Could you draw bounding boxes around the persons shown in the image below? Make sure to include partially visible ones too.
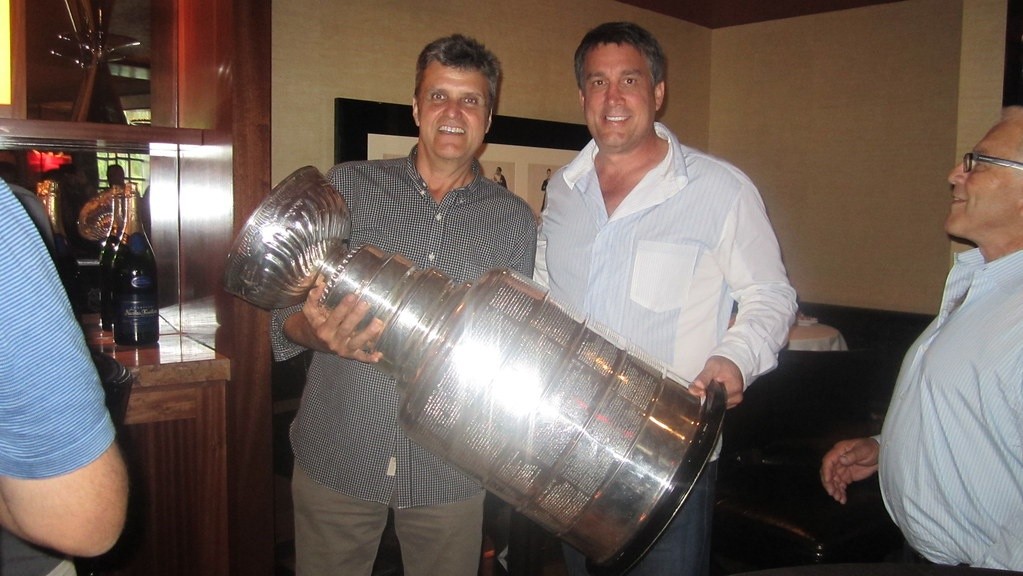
[106,163,127,189]
[822,104,1023,576]
[270,33,538,576]
[531,20,798,576]
[0,174,129,557]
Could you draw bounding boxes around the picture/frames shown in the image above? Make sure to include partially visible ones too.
[333,97,593,221]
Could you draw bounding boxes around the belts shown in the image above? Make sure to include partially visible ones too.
[903,542,932,564]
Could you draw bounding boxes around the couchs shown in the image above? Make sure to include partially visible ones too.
[710,301,953,573]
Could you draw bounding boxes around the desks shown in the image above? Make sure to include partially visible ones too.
[727,313,848,353]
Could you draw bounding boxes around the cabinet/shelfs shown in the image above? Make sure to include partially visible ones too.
[0,0,272,576]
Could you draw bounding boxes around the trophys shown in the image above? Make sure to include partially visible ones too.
[223,164,727,576]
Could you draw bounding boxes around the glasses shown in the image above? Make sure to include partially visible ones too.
[963,153,1023,174]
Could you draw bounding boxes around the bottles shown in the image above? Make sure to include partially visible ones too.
[44,181,80,323]
[113,182,160,346]
[101,185,124,332]
[37,183,45,208]
